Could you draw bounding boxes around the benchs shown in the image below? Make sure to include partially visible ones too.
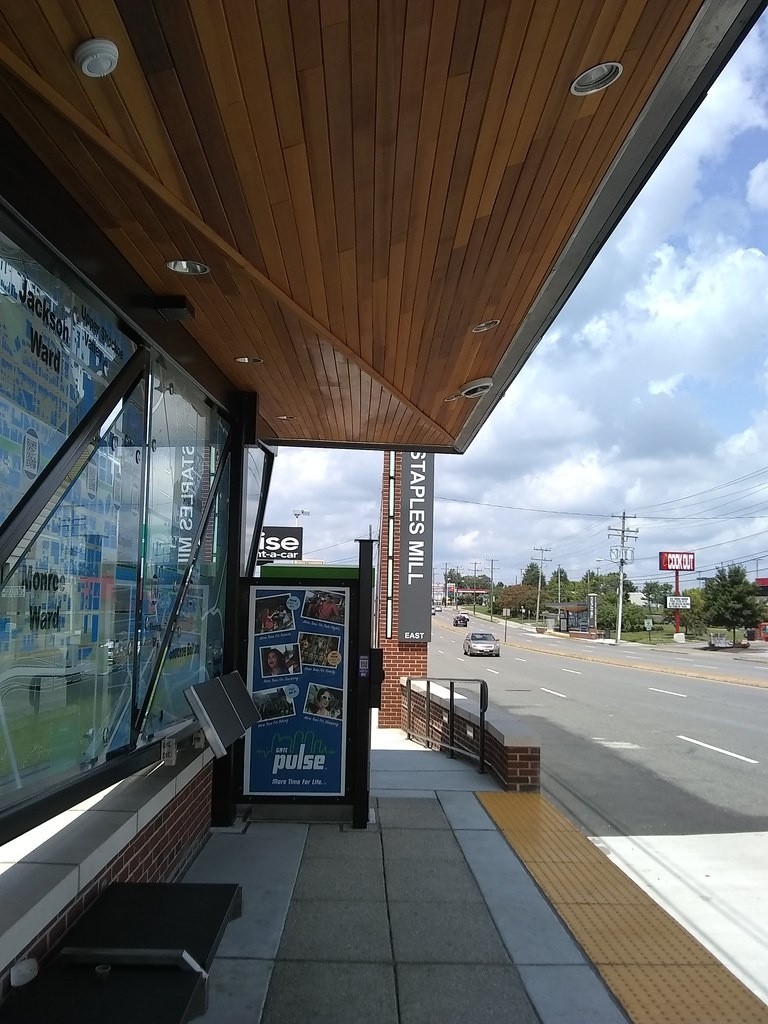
[0,881,244,1024]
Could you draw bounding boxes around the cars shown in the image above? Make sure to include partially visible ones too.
[453,611,469,626]
[463,633,501,657]
[431,605,442,615]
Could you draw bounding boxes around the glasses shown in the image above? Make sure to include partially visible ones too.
[320,695,332,701]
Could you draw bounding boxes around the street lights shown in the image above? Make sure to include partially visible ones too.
[596,559,624,643]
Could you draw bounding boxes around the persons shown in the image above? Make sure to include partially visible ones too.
[308,689,341,718]
[316,595,340,619]
[267,649,290,676]
[278,605,288,624]
[306,597,320,617]
[263,608,278,631]
